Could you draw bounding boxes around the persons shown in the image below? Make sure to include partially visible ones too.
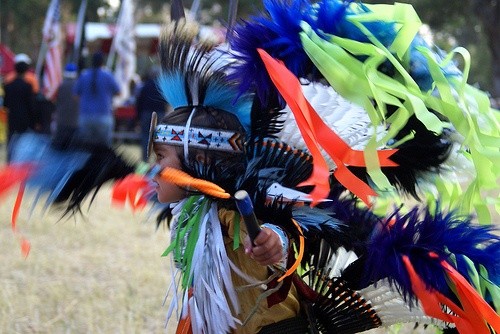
[52,63,82,148]
[75,52,120,146]
[3,53,44,165]
[148,104,315,333]
